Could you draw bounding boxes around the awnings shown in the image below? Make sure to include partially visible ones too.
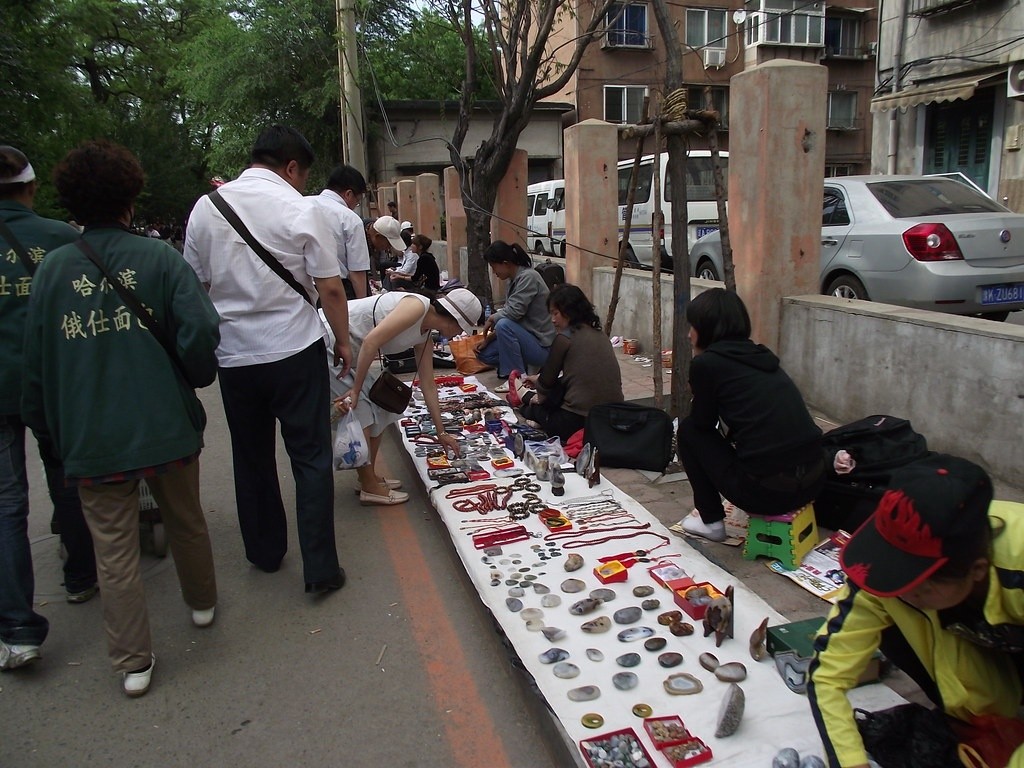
[869,69,1008,113]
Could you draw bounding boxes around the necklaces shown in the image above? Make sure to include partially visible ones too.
[544,487,672,549]
[501,490,512,510]
[493,493,500,510]
[445,484,497,514]
[460,516,519,533]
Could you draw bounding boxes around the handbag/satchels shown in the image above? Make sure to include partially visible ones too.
[448,333,495,376]
[369,371,412,414]
[333,407,371,471]
[582,401,673,473]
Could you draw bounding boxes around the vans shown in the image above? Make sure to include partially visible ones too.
[546,150,731,268]
[526,179,565,257]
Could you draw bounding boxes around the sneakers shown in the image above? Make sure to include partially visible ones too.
[0,639,41,669]
[66,582,98,603]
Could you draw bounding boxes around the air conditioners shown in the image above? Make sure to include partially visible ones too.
[704,49,726,69]
[868,42,877,56]
[1007,64,1024,97]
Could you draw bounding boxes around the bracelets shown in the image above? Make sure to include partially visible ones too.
[529,504,548,513]
[507,477,544,512]
[509,510,530,520]
[493,486,508,494]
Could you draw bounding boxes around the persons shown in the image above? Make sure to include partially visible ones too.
[474,241,557,391]
[681,287,824,539]
[507,282,624,441]
[0,146,98,666]
[137,216,188,254]
[319,287,483,505]
[304,165,370,301]
[812,452,1024,768]
[184,124,350,591]
[29,143,220,697]
[363,202,440,303]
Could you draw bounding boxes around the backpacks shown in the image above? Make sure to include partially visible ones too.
[817,414,941,499]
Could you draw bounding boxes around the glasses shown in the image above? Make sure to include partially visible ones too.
[347,188,361,207]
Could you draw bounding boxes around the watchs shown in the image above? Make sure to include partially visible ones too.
[437,431,448,438]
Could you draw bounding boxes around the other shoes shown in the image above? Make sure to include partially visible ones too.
[494,384,510,393]
[192,606,215,627]
[123,652,156,695]
[359,489,409,505]
[506,369,521,407]
[355,476,401,495]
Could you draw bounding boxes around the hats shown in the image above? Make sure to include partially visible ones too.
[400,221,413,231]
[436,288,482,337]
[373,216,407,251]
[839,456,994,598]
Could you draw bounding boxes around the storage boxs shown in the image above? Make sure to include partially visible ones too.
[673,581,725,620]
[491,456,514,470]
[644,715,692,751]
[593,560,628,585]
[662,736,713,768]
[427,457,450,469]
[462,384,479,392]
[539,512,572,533]
[580,727,657,768]
[766,617,886,689]
[401,420,422,438]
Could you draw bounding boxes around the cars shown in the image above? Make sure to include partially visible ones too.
[690,172,1024,324]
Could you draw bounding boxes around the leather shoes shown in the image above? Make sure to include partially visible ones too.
[306,567,345,593]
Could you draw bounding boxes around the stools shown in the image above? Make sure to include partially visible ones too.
[742,502,819,571]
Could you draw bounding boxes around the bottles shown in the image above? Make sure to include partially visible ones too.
[330,397,351,424]
[441,334,448,345]
[485,305,491,321]
